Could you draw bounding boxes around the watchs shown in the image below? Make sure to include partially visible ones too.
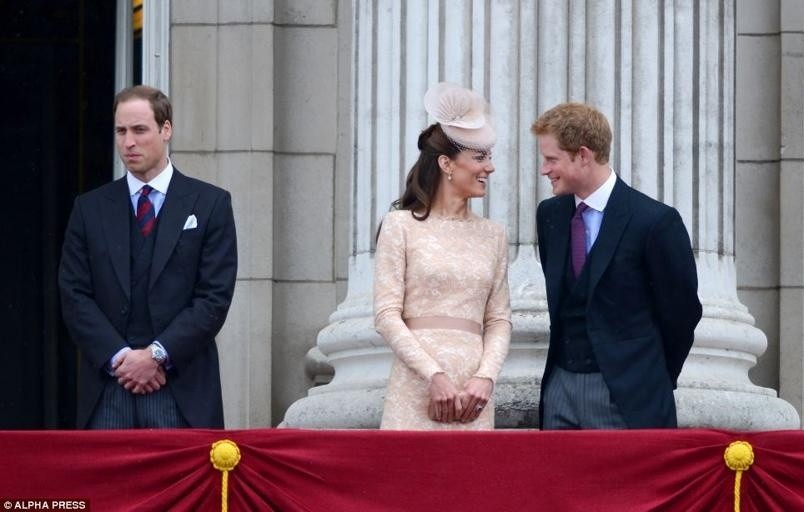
[148,342,165,365]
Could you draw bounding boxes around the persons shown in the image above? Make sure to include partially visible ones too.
[529,101,702,428]
[56,87,238,430]
[371,82,513,430]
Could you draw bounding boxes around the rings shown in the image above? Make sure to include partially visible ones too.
[475,403,482,413]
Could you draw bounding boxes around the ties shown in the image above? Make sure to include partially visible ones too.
[136,185,157,239]
[569,201,588,281]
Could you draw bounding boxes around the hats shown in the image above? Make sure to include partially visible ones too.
[440,115,498,153]
